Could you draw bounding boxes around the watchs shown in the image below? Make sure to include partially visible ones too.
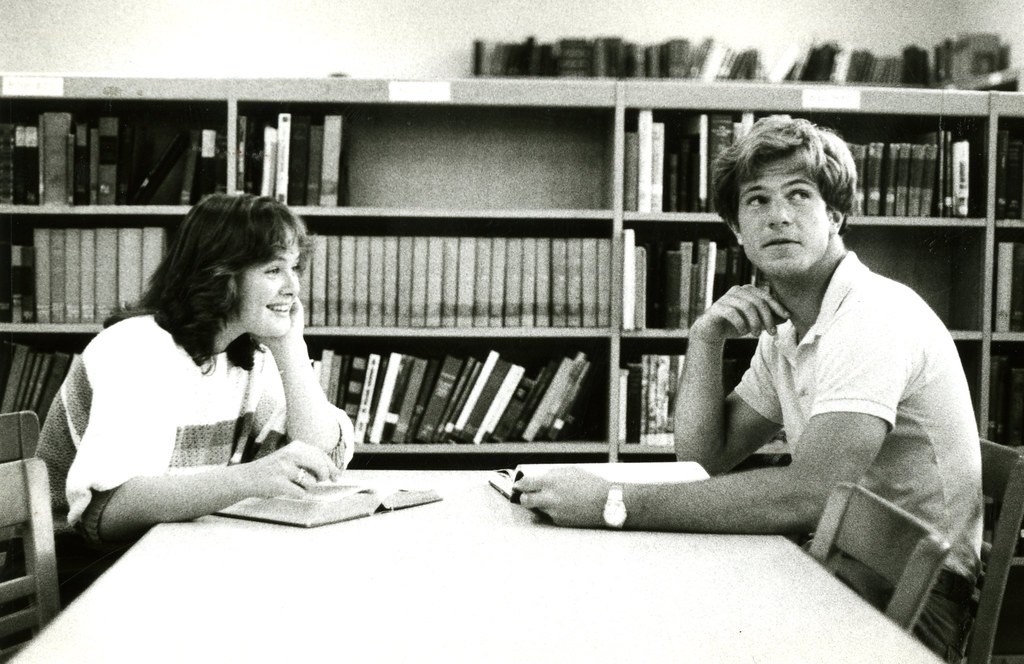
[603,485,628,528]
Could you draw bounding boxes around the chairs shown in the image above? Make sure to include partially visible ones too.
[0,410,64,662]
[806,435,1024,660]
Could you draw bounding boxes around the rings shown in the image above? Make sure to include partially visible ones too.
[295,469,309,484]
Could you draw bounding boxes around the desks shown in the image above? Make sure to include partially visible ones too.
[4,460,943,663]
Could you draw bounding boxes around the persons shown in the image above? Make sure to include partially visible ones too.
[0,192,357,646]
[513,111,985,664]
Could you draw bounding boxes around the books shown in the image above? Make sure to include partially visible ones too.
[0,33,1024,441]
[212,482,441,529]
[486,461,711,500]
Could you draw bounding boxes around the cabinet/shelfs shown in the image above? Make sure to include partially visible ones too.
[0,72,1024,647]
